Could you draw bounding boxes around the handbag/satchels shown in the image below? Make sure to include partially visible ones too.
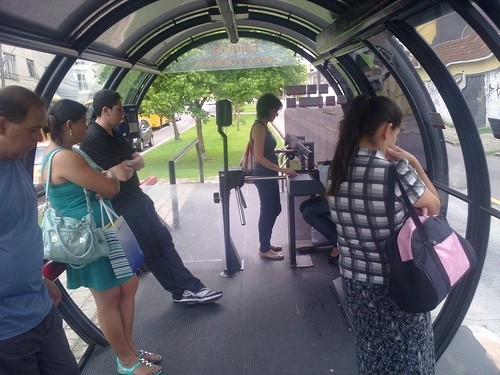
[385,161,479,313]
[239,125,257,184]
[40,151,109,264]
[98,197,146,280]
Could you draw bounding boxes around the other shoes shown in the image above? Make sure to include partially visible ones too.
[259,250,284,260]
[328,251,339,264]
[271,246,282,251]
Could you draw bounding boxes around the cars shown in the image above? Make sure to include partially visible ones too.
[131,119,154,152]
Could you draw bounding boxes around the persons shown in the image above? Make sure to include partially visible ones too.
[250,93,297,260]
[325,94,441,375]
[42,99,163,375]
[299,161,339,261]
[0,85,81,375]
[78,89,223,304]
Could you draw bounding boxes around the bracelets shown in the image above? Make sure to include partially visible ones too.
[41,274,46,279]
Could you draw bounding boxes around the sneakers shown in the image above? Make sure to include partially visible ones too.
[173,286,223,304]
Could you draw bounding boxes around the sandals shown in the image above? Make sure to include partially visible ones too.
[116,348,163,375]
